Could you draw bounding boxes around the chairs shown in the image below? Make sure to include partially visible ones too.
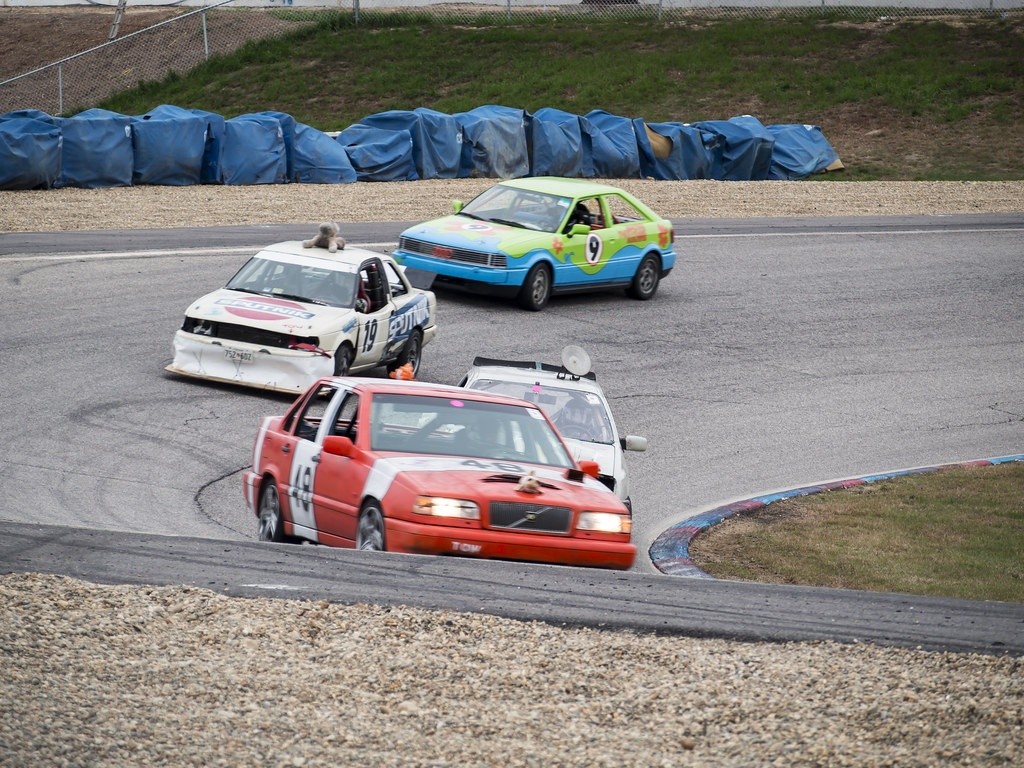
[556,398,597,439]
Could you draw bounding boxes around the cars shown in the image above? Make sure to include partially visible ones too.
[242,376,637,571]
[418,356,648,520]
[164,241,438,400]
[390,176,677,310]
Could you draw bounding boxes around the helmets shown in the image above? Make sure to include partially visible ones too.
[565,204,590,230]
[466,419,500,445]
[335,270,362,289]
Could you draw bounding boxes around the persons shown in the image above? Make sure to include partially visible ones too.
[460,417,518,458]
[324,273,371,313]
[550,200,591,233]
[554,399,596,442]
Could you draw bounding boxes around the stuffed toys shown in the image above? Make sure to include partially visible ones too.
[303,221,345,253]
